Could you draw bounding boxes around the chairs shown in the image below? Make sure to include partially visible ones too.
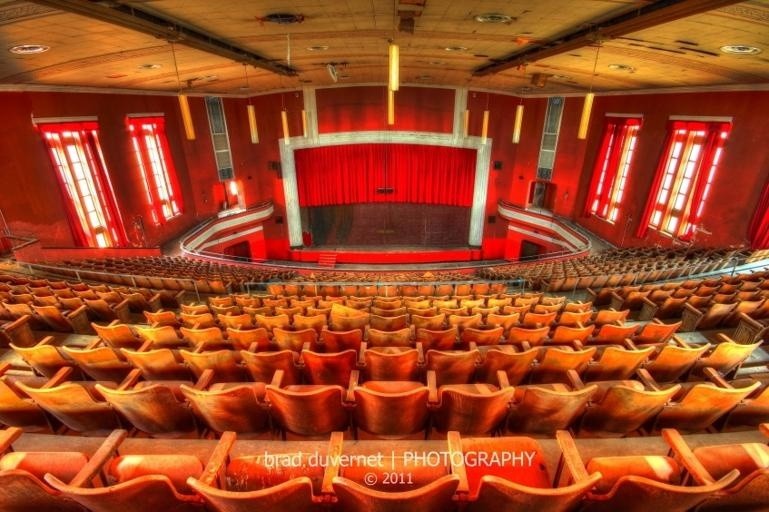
[693,423,769,512]
[1,426,128,512]
[44,430,238,512]
[331,431,469,511]
[460,429,602,511]
[583,428,741,512]
[186,430,344,511]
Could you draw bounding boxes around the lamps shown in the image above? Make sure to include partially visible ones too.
[280,78,290,145]
[387,91,395,125]
[577,46,602,139]
[511,66,527,144]
[243,64,260,145]
[463,110,470,137]
[169,42,197,140]
[480,73,492,144]
[389,44,400,91]
